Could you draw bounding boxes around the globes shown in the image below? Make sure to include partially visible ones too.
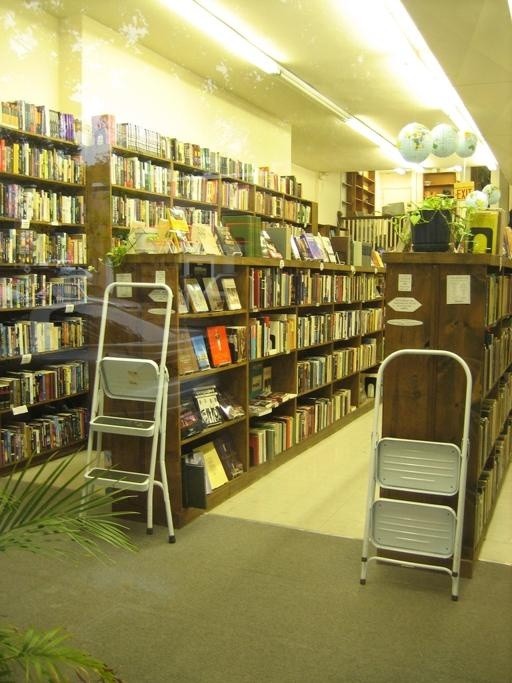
[397,122,477,163]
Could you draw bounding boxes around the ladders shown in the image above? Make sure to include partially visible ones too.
[76,282,176,543]
[361,348,472,601]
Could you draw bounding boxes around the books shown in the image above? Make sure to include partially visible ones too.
[1,100,89,462]
[474,271,512,556]
[177,215,386,509]
[462,206,507,258]
[112,123,312,249]
